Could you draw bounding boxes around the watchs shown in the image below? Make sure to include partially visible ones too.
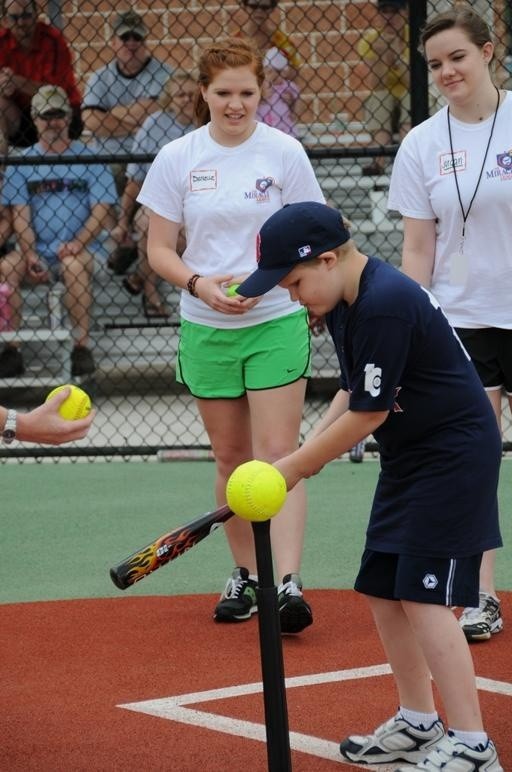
[2,409,17,439]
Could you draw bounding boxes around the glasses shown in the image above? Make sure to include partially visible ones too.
[119,32,142,41]
[40,111,65,120]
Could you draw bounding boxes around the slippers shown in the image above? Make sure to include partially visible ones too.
[109,246,168,317]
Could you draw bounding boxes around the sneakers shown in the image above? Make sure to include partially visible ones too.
[277,574,313,633]
[0,346,25,378]
[71,345,96,384]
[458,592,503,640]
[213,567,258,622]
[340,713,504,772]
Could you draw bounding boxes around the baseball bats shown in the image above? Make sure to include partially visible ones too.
[110,503,235,590]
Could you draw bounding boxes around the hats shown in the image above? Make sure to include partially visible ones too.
[112,11,147,38]
[31,85,73,116]
[235,201,350,298]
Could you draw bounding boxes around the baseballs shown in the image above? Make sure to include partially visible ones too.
[227,284,241,297]
[226,460,286,521]
[46,384,91,419]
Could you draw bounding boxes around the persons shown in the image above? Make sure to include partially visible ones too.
[0,385,97,445]
[236,202,504,772]
[356,2,412,176]
[387,6,511,643]
[0,0,307,384]
[136,39,328,632]
